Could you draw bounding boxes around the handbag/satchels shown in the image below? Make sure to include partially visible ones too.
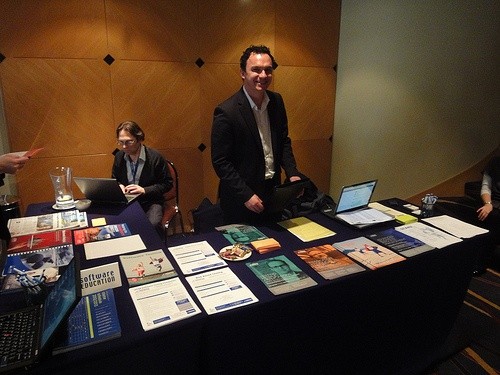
[192,197,226,233]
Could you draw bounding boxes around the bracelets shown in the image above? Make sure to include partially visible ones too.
[484,201,491,204]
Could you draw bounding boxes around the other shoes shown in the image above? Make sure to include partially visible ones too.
[473,270,478,277]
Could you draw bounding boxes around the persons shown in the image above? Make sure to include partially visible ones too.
[111,120,174,242]
[464,155,500,277]
[266,259,309,288]
[354,247,368,256]
[210,45,305,227]
[361,243,391,257]
[131,261,145,278]
[0,151,31,188]
[219,225,250,242]
[150,257,164,272]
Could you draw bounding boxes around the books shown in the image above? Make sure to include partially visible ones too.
[0,202,489,356]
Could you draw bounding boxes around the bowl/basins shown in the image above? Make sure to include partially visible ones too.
[73,200,91,210]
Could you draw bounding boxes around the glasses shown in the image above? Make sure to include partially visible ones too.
[117,139,137,145]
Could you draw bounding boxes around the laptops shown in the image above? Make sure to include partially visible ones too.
[73,177,141,207]
[334,179,395,230]
[0,253,82,374]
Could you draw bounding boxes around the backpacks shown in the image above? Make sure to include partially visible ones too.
[281,172,335,222]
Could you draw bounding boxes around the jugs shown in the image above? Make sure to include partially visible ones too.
[49,167,74,207]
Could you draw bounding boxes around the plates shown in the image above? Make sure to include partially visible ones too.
[52,200,79,210]
[219,246,252,261]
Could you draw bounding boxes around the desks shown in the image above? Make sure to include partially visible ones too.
[22,195,479,375]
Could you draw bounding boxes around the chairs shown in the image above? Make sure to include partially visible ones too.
[154,159,184,250]
